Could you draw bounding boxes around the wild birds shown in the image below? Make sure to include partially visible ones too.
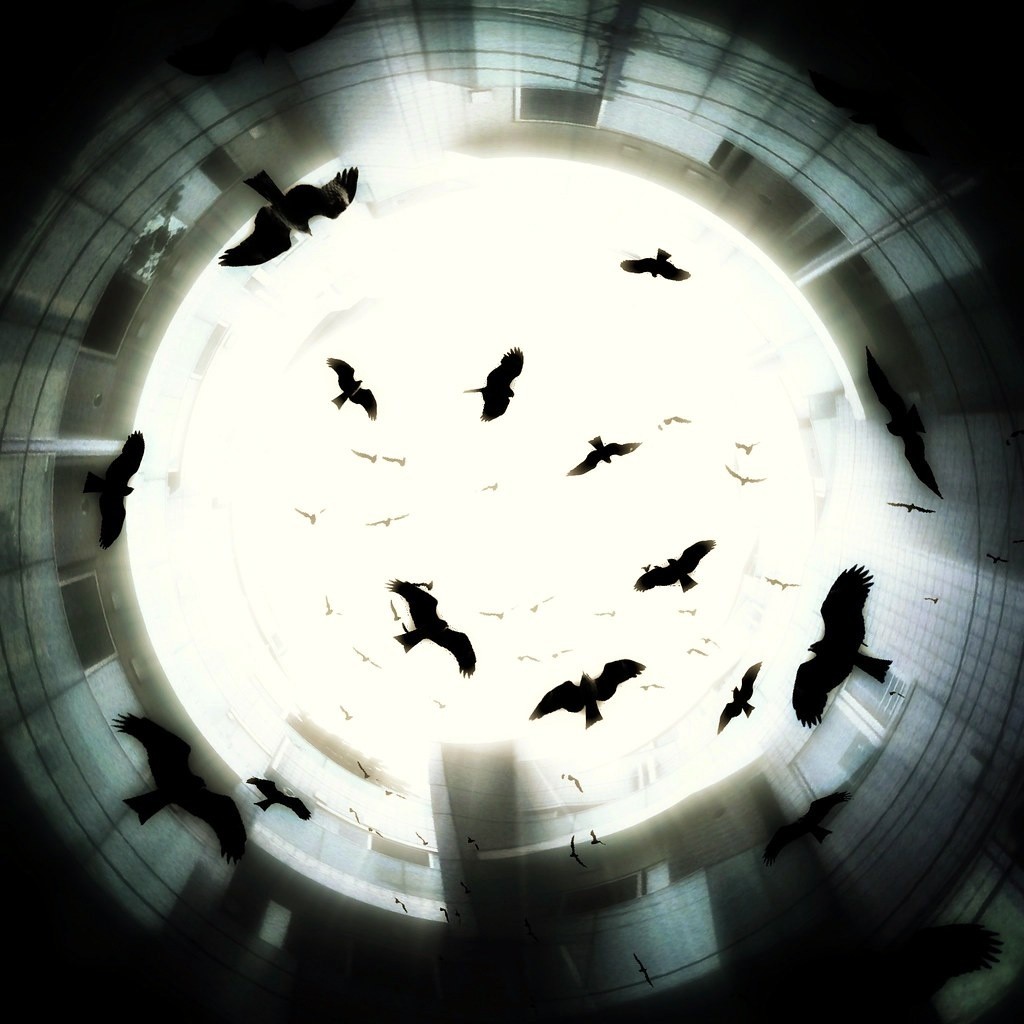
[81,167,1019,942]
